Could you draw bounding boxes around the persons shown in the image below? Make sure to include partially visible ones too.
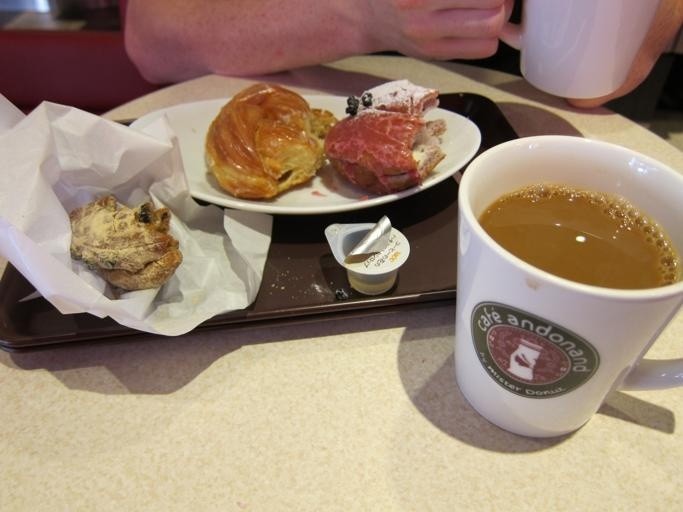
[119,0,683,108]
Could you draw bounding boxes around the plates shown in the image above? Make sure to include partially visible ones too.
[122,92,484,219]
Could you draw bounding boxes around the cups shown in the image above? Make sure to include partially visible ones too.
[452,135,683,444]
[496,1,665,104]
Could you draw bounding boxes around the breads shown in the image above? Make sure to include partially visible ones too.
[204,83,337,200]
[71,196,181,291]
[324,76,446,194]
